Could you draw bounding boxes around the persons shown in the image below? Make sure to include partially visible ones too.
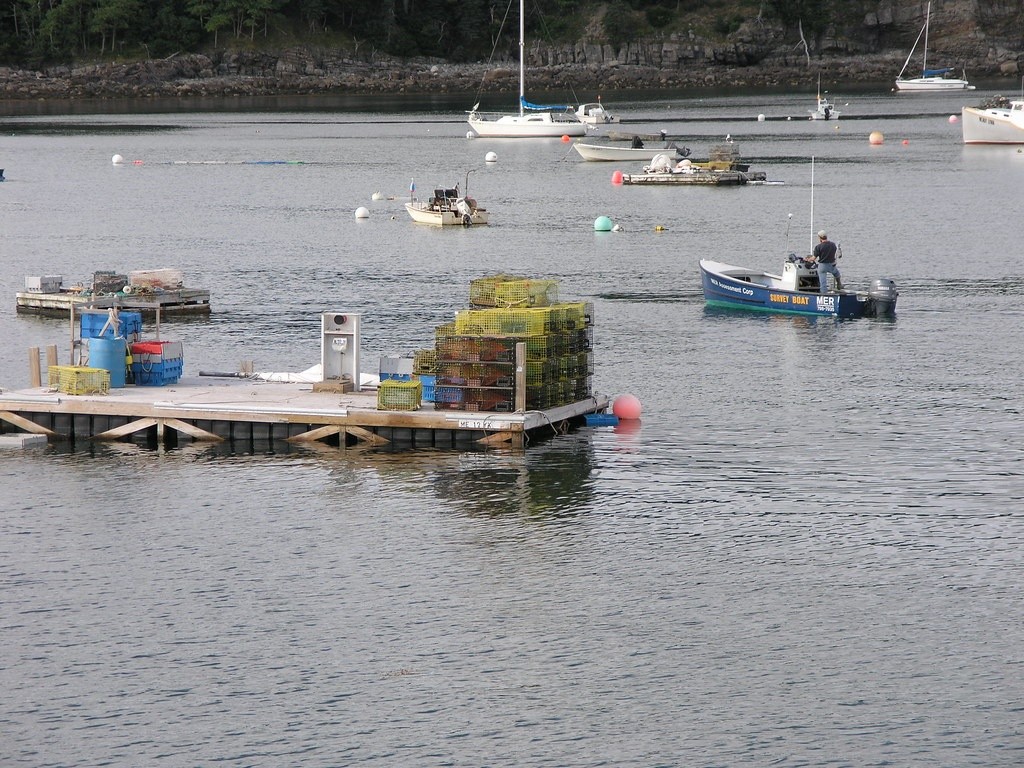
[803,230,844,293]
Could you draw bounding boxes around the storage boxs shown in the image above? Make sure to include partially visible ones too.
[375,273,594,412]
[47,364,112,395]
[130,341,183,387]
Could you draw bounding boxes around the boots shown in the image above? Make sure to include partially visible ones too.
[836,277,844,290]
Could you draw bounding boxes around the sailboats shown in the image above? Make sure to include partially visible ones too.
[463,0,590,138]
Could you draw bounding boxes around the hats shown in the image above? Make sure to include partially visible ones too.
[818,230,827,238]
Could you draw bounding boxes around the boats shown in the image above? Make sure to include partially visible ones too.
[697,155,898,321]
[961,98,1024,144]
[573,138,693,161]
[690,157,751,172]
[894,1,977,90]
[574,100,620,124]
[812,97,841,121]
[605,128,667,140]
[403,178,490,227]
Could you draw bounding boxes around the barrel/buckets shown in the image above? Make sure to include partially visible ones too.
[89,335,126,388]
